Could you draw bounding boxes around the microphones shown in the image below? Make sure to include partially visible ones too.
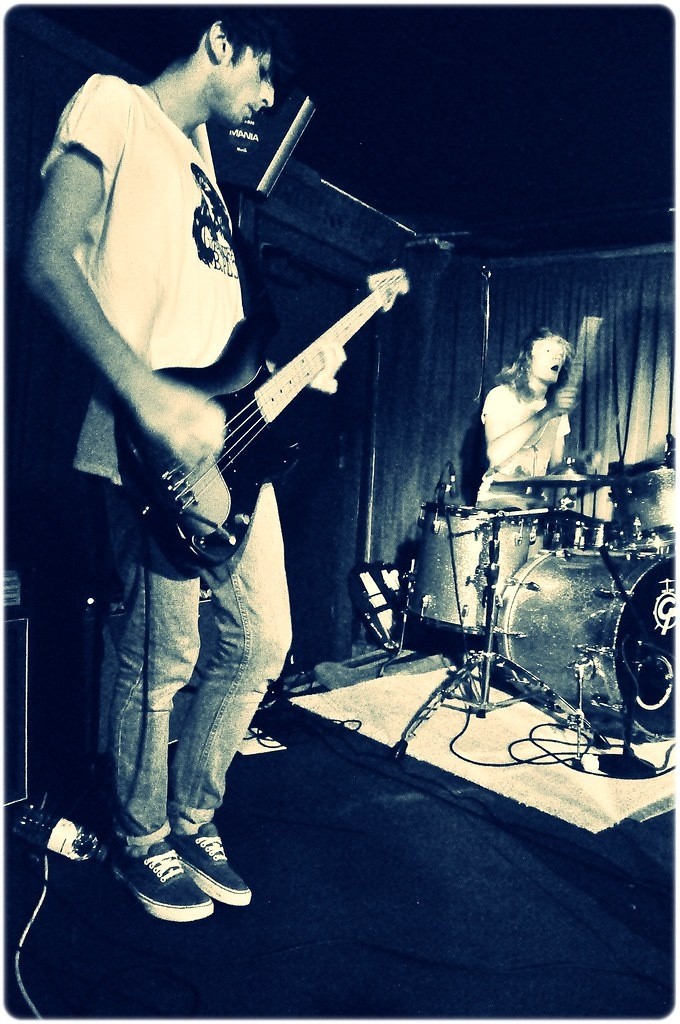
[448,463,458,499]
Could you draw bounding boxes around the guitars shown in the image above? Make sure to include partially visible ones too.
[121,267,410,571]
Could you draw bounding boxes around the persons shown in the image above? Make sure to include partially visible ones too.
[479,327,603,511]
[24,4,345,923]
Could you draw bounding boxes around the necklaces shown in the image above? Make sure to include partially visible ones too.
[145,82,164,112]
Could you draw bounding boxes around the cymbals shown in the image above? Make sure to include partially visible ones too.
[489,468,619,490]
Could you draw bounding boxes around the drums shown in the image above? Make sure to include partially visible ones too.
[496,545,675,743]
[610,467,675,553]
[408,500,533,642]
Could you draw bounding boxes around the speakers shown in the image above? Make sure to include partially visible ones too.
[4,604,38,808]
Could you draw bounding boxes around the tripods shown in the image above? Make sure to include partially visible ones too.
[388,482,610,767]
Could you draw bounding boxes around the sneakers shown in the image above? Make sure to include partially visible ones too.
[169,822,254,907]
[112,842,215,924]
